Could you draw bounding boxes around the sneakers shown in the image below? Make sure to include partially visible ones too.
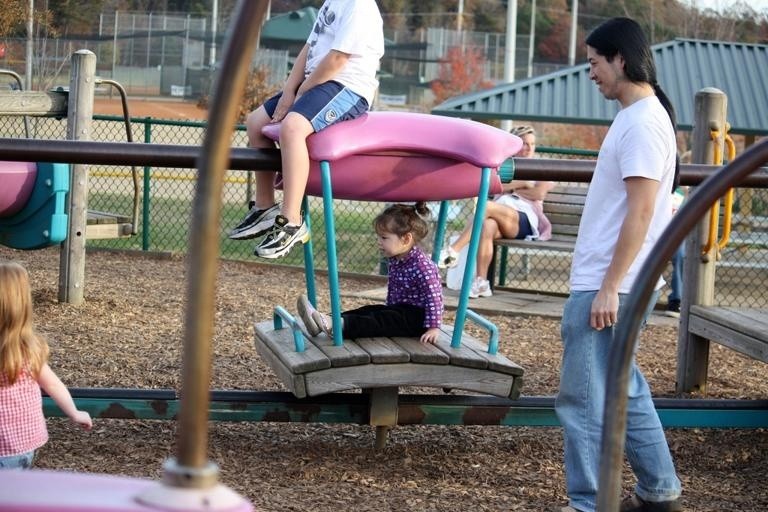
[427,244,462,268]
[618,494,687,512]
[227,202,281,242]
[295,292,342,340]
[254,211,313,261]
[466,275,492,298]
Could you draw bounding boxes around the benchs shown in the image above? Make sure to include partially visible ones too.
[486,181,588,293]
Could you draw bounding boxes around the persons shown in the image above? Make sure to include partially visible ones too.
[438,126,552,299]
[1,260,93,469]
[552,16,683,512]
[668,189,685,311]
[226,0,386,261]
[297,202,445,344]
